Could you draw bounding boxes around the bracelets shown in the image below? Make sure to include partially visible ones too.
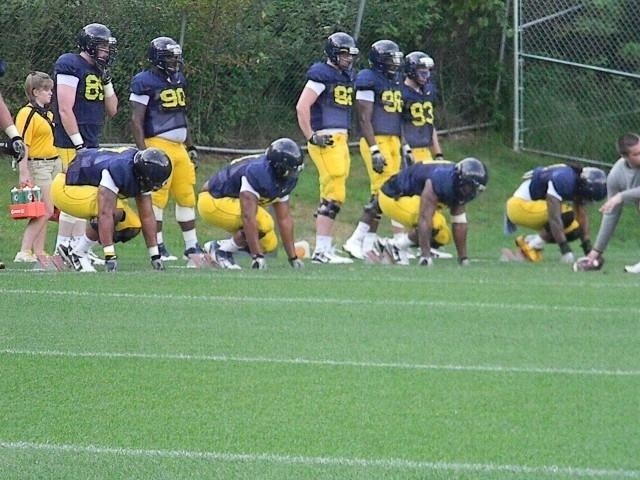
[558,243,571,253]
[582,240,592,254]
[592,248,603,253]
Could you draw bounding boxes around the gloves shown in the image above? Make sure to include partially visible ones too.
[0,138,15,158]
[401,150,416,169]
[185,145,202,170]
[4,135,29,164]
[248,253,270,272]
[95,56,113,86]
[457,256,473,267]
[578,239,605,271]
[418,256,436,268]
[434,153,447,162]
[371,149,388,174]
[559,241,576,266]
[287,255,307,271]
[148,254,166,271]
[308,131,337,149]
[72,143,88,153]
[102,252,120,274]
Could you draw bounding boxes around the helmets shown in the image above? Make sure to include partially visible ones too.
[454,156,488,192]
[323,30,361,60]
[146,34,185,66]
[76,22,119,52]
[134,147,173,193]
[402,50,436,74]
[580,164,608,202]
[367,37,405,69]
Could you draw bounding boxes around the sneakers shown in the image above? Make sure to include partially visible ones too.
[65,249,99,275]
[623,259,640,276]
[310,247,358,267]
[11,248,40,264]
[0,259,6,270]
[52,249,67,265]
[416,245,455,260]
[33,251,53,267]
[342,237,417,268]
[157,241,179,265]
[86,247,107,268]
[56,240,73,260]
[183,242,214,270]
[211,247,246,273]
[203,239,222,257]
[514,234,544,263]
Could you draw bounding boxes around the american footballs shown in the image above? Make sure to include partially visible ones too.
[573,256,604,272]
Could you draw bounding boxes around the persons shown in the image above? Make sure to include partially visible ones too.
[575,132,640,273]
[506,162,606,270]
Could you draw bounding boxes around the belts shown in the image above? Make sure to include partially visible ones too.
[26,154,63,162]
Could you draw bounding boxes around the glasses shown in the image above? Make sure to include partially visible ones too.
[264,136,305,173]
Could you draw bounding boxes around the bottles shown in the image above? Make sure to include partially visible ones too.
[11,185,41,204]
